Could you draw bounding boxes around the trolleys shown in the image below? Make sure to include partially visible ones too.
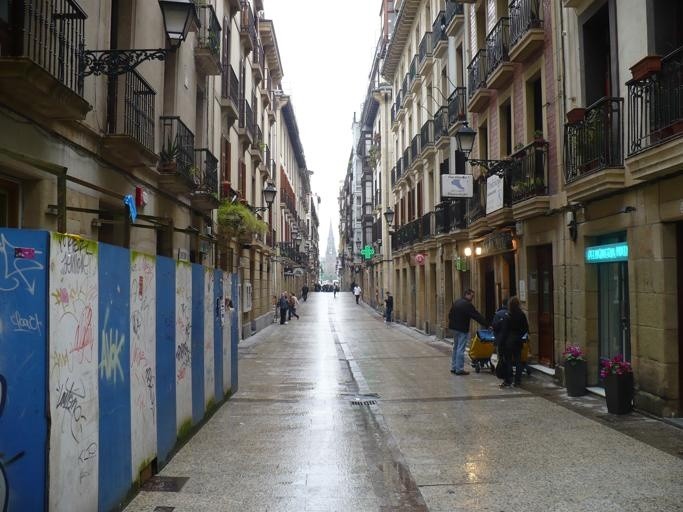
[468,327,495,373]
[512,330,532,376]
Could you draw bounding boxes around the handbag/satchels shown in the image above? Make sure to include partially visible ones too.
[520,336,529,362]
[495,352,513,379]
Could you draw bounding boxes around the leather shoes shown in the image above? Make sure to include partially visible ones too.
[456,370,469,375]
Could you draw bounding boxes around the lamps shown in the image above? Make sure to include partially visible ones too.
[383,207,415,230]
[274,226,302,250]
[246,181,277,214]
[82,0,197,88]
[455,121,522,178]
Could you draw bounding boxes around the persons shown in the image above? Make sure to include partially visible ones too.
[382,291,393,324]
[447,289,487,376]
[491,297,530,377]
[301,280,362,305]
[489,296,529,388]
[276,290,300,325]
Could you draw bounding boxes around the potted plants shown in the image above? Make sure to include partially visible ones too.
[218,203,270,247]
[160,134,181,174]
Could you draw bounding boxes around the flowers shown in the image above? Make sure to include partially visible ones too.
[600,354,632,377]
[561,344,587,362]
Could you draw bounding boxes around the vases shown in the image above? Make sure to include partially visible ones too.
[564,360,587,396]
[601,374,634,414]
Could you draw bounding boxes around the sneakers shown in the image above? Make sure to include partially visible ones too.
[499,382,510,389]
